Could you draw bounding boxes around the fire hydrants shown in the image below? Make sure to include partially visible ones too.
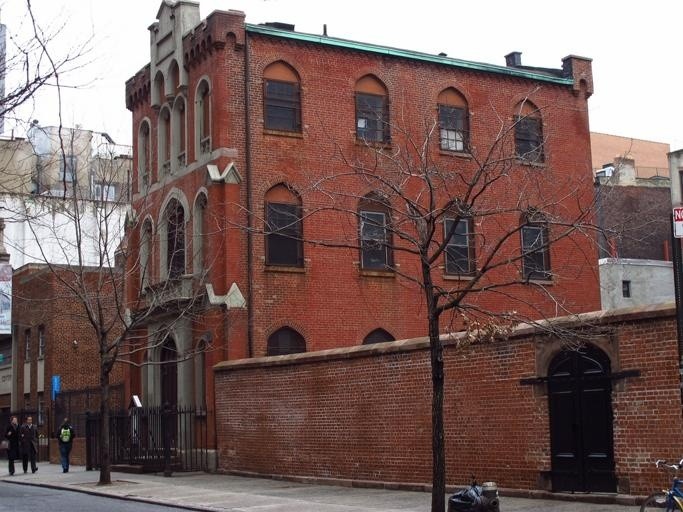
[473,482,500,512]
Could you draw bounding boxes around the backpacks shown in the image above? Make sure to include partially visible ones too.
[60,428,70,442]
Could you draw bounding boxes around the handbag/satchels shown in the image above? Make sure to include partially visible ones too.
[0,440,10,450]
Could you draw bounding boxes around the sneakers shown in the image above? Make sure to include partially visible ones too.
[32,467,38,473]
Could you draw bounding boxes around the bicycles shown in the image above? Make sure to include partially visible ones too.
[640,459,683,512]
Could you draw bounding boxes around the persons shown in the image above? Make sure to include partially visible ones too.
[5,416,24,475]
[20,416,39,473]
[56,417,75,473]
